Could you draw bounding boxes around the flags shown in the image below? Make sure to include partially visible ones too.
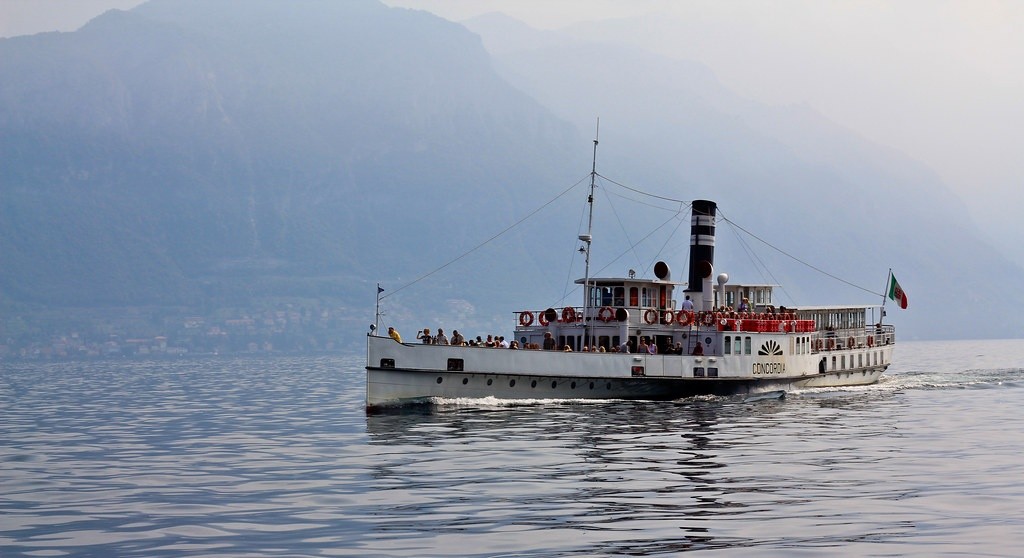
[888,272,908,310]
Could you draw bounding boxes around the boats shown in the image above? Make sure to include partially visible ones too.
[364,116,896,412]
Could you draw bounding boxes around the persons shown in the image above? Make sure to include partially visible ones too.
[714,298,797,320]
[388,327,402,343]
[584,338,704,356]
[682,295,694,311]
[418,328,571,351]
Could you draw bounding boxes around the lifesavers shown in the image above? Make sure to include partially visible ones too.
[723,312,730,318]
[792,313,798,320]
[599,306,614,322]
[811,340,814,349]
[775,313,790,320]
[734,312,740,319]
[715,312,723,325]
[663,311,674,324]
[849,337,855,347]
[520,311,534,326]
[816,339,823,352]
[741,312,758,319]
[562,307,575,323]
[677,310,690,326]
[702,311,715,326]
[644,309,658,325]
[759,312,774,320]
[868,336,873,347]
[828,339,834,350]
[539,312,549,326]
